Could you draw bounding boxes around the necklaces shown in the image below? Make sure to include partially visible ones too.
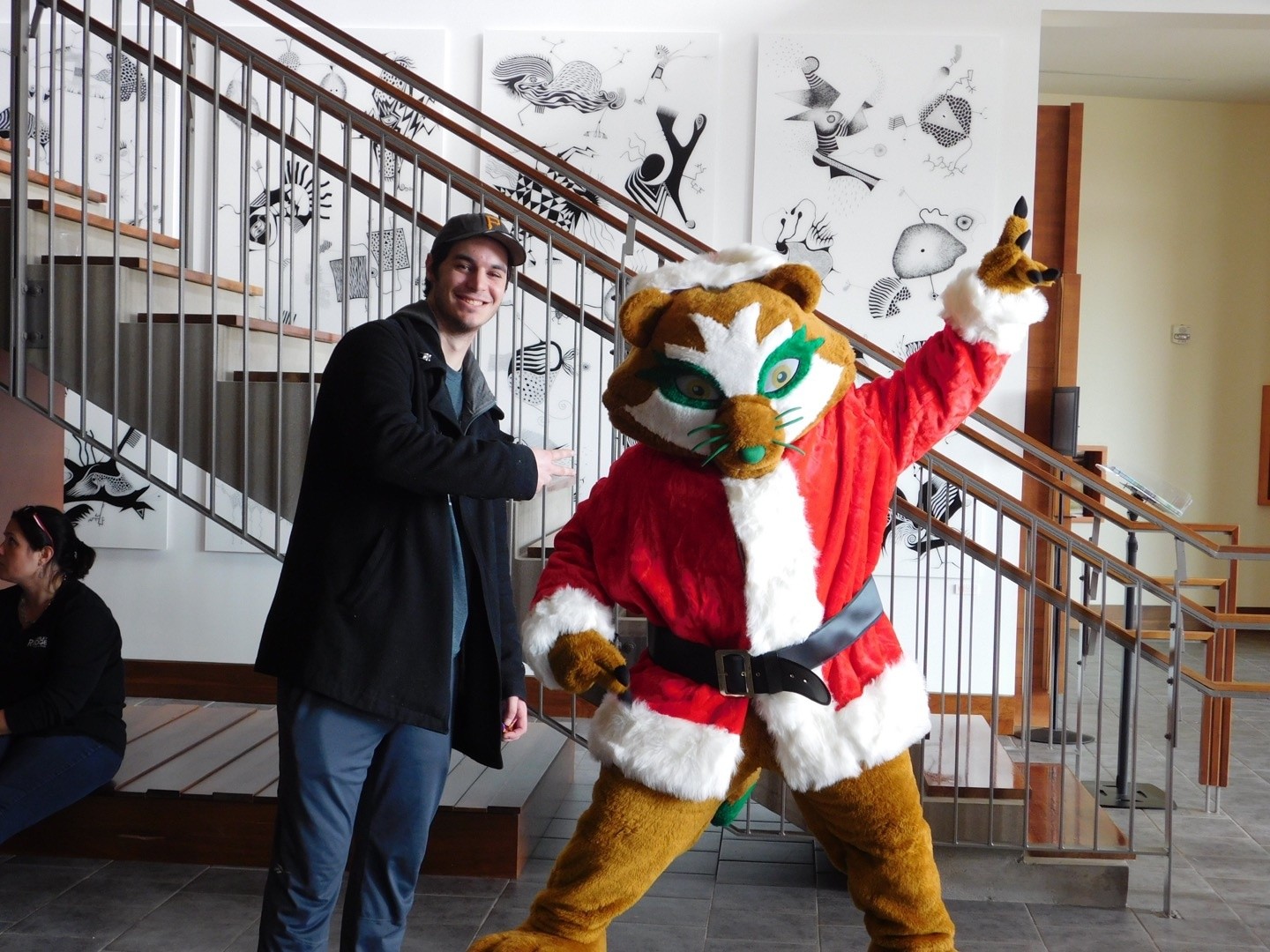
[18,573,67,626]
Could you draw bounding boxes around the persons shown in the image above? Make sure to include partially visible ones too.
[0,502,127,845]
[258,211,598,951]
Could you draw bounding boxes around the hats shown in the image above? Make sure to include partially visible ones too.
[432,212,527,266]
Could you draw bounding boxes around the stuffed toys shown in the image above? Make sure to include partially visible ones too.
[468,193,1062,951]
